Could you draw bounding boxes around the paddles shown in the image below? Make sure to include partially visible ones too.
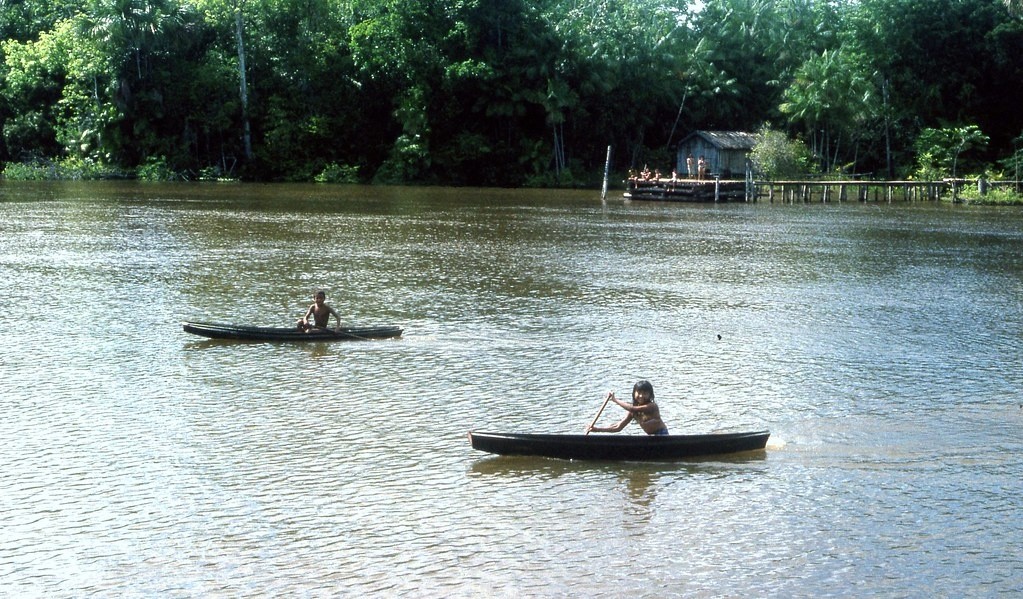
[584,392,614,436]
[300,324,371,341]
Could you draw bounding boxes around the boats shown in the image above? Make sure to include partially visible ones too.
[185,321,404,341]
[467,431,773,458]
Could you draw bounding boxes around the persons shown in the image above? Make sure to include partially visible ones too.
[687,154,697,181]
[698,155,706,181]
[666,168,681,192]
[628,163,662,189]
[587,380,668,436]
[296,291,342,335]
